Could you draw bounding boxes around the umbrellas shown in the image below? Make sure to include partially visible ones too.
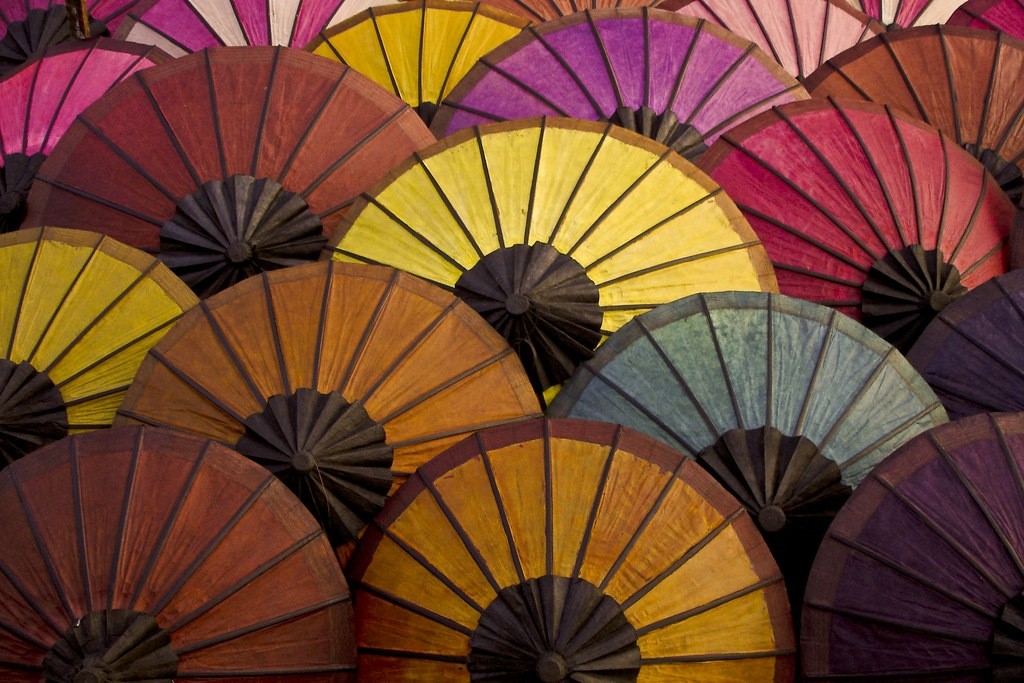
[0,1,1024,612]
[0,426,362,683]
[800,410,1024,683]
[343,416,797,683]
